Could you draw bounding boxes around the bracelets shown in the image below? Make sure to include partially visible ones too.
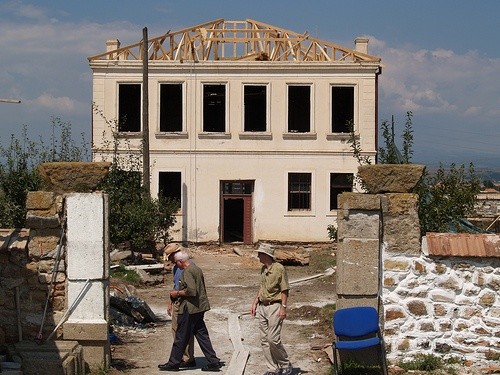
[177,291,179,295]
[283,305,286,307]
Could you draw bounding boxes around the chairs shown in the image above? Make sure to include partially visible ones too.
[333,307,388,375]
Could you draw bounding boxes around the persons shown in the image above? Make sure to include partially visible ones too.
[164,243,196,367]
[251,243,293,375]
[158,252,222,371]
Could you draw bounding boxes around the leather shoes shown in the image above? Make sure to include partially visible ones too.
[202,361,222,371]
[158,363,179,370]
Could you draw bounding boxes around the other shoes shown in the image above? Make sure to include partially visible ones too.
[179,359,196,368]
[278,362,293,375]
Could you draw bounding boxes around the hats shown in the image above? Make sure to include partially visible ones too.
[255,243,275,259]
[164,243,183,261]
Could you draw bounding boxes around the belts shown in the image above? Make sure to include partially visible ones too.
[259,300,282,304]
